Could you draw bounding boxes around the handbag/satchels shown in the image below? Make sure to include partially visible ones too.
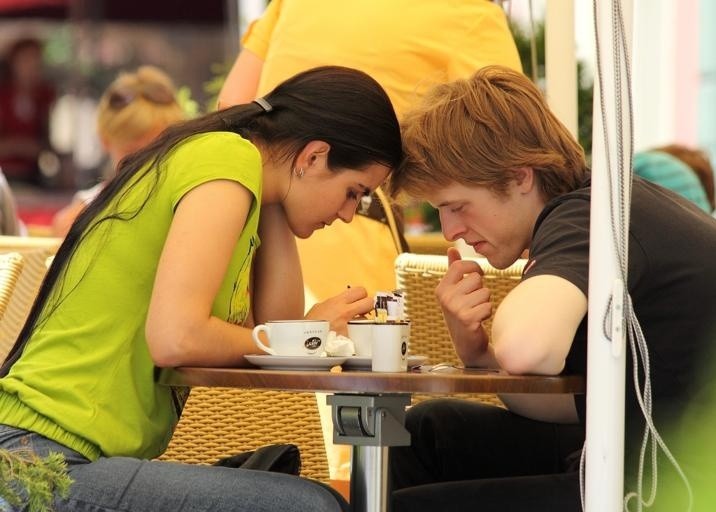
[213,444,300,475]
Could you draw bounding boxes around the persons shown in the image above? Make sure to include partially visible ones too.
[632,138,714,218]
[0,32,58,187]
[50,63,193,236]
[383,62,714,511]
[211,1,522,504]
[0,63,404,512]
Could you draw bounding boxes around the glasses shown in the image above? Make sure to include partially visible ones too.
[110,83,172,107]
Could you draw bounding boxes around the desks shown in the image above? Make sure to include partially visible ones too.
[156,367,586,512]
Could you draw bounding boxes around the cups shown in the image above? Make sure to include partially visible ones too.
[371,322,410,374]
[347,319,378,356]
[251,318,330,356]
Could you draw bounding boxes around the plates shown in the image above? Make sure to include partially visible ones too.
[341,355,428,371]
[243,353,349,371]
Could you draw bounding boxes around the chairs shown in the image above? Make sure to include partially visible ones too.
[0,235,330,487]
[394,254,532,413]
